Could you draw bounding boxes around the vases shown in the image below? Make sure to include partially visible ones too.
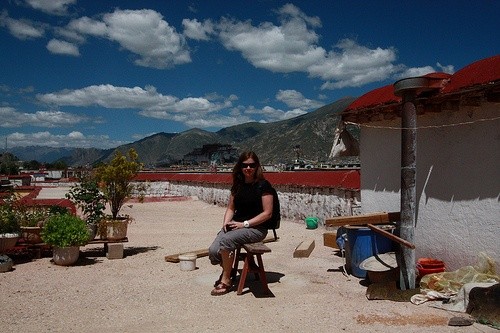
[99,217,128,240]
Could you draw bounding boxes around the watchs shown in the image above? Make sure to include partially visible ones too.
[243,221,249,228]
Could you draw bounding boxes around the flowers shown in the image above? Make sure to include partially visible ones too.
[100,151,142,218]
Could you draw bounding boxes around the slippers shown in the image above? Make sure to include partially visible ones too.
[210,281,236,296]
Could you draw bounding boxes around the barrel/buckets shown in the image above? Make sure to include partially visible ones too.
[416,259,445,277]
[345,227,397,278]
[178,252,197,271]
[305,216,318,229]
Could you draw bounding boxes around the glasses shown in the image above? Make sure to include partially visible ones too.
[240,163,257,168]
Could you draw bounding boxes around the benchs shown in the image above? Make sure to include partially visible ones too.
[234,241,271,295]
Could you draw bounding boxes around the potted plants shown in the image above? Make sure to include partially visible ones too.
[64,167,104,240]
[0,203,19,253]
[20,205,50,242]
[42,213,88,264]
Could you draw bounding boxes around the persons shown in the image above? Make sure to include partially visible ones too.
[208,150,273,296]
